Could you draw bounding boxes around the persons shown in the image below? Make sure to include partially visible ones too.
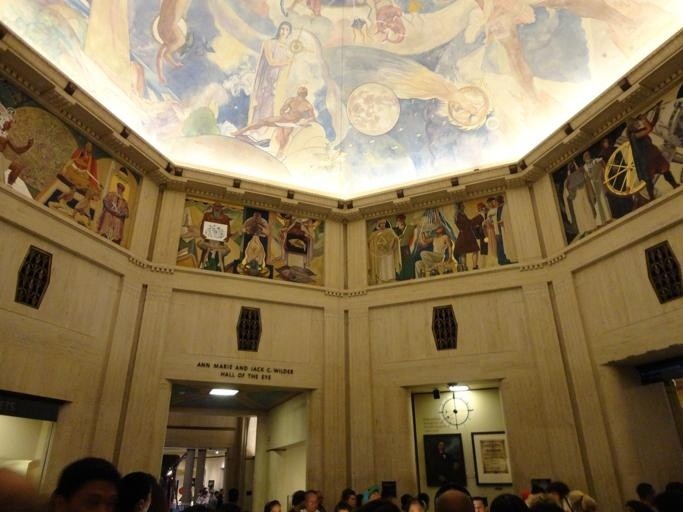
[626,100,680,202]
[56,140,104,218]
[596,137,633,219]
[95,183,128,246]
[199,200,231,270]
[231,87,314,159]
[0,113,33,186]
[366,195,518,285]
[581,151,614,227]
[247,22,302,144]
[563,160,604,236]
[425,436,460,485]
[241,210,269,271]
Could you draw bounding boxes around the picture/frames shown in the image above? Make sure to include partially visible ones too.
[422,432,470,488]
[471,431,513,486]
